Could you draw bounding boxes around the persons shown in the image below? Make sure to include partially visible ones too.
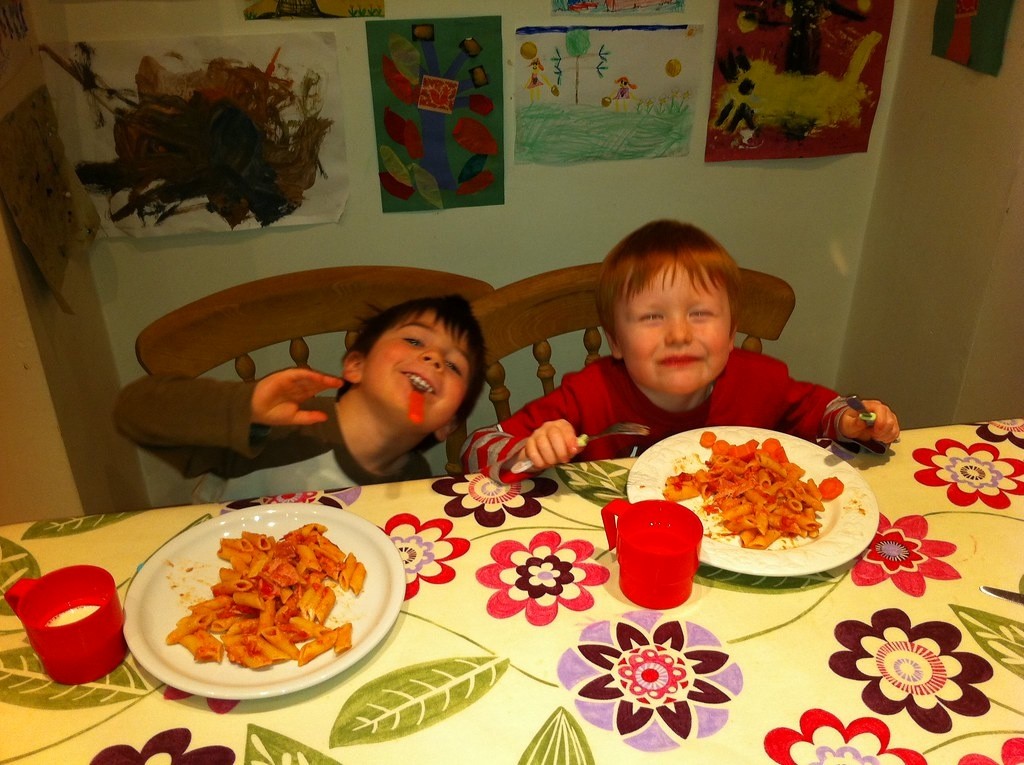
[111,293,490,507]
[459,218,900,486]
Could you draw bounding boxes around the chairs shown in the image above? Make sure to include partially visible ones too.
[472,259,797,421]
[133,265,496,476]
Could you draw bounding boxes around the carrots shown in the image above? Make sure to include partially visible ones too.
[699,431,844,499]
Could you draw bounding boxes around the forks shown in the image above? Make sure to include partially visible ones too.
[407,379,427,425]
[509,421,650,474]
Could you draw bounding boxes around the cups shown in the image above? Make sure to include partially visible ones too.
[4,564,126,683]
[602,500,702,610]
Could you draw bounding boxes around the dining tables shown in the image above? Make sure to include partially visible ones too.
[0,418,1024,765]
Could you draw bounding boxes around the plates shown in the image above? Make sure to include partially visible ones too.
[626,426,880,576]
[122,502,406,700]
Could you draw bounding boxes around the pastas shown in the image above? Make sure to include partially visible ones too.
[165,523,365,670]
[663,455,825,548]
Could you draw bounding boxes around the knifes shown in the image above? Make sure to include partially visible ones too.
[845,395,901,443]
